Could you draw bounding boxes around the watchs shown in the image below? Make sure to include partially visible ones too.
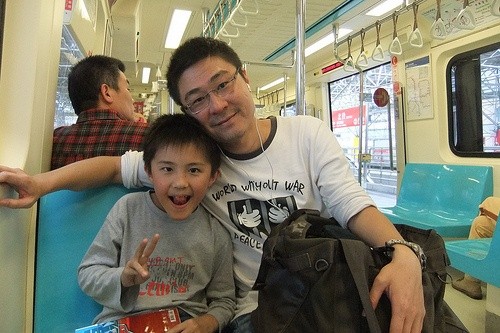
[384,239,428,268]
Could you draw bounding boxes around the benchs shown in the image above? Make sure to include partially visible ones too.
[444,212,500,333]
[376,163,493,238]
[34,185,154,333]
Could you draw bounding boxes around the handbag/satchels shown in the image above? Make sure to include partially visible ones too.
[249,208,454,333]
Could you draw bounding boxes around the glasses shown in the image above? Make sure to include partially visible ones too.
[184,66,242,115]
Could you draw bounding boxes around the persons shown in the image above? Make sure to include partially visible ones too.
[51,55,153,170]
[451,197,500,300]
[78,113,237,332]
[1,37,428,333]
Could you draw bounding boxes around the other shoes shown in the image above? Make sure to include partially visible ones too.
[452,277,483,300]
